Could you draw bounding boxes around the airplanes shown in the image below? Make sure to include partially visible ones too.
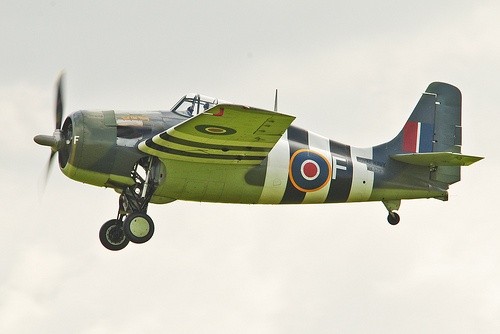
[31,70,488,253]
